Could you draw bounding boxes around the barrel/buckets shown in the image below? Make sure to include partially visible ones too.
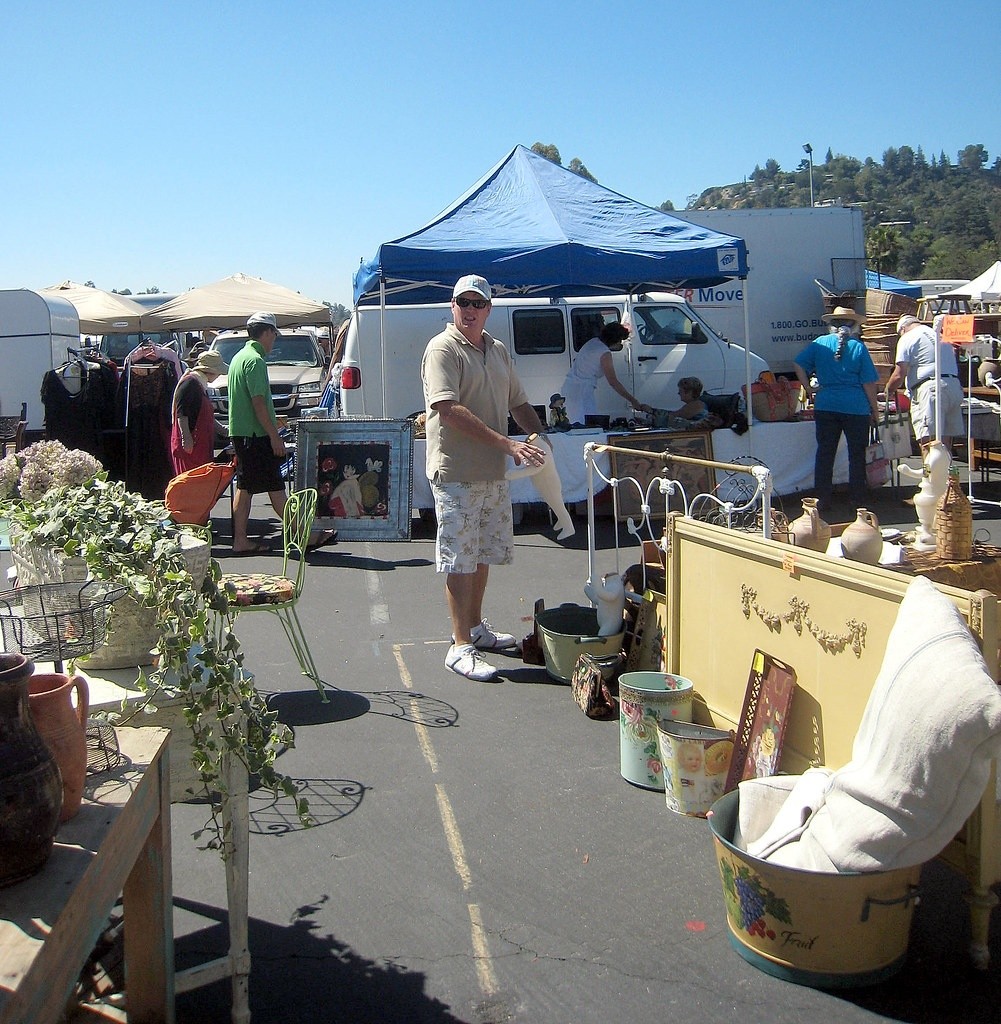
[616,671,737,819]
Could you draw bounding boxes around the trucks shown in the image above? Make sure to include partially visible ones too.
[84,294,186,378]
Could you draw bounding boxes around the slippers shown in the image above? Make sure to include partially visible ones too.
[231,543,274,556]
[306,528,338,552]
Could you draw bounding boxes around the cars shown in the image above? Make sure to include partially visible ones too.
[206,329,331,437]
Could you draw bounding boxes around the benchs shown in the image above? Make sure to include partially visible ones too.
[0,403,31,458]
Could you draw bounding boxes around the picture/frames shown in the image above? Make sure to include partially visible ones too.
[293,417,414,543]
[606,428,719,521]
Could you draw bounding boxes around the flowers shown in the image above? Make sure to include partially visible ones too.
[0,439,103,507]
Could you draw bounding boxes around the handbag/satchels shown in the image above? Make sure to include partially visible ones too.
[571,653,622,720]
[865,423,893,489]
[741,376,801,421]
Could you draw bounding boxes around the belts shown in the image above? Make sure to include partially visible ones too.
[910,374,958,391]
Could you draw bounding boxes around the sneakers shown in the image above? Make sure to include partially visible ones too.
[450,617,517,648]
[444,644,499,681]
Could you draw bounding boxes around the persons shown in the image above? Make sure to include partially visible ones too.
[548,394,570,429]
[794,305,879,512]
[884,314,964,507]
[170,349,229,475]
[228,312,338,558]
[420,273,554,682]
[638,377,709,422]
[562,321,643,427]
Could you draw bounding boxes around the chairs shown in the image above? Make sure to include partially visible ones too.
[212,488,331,704]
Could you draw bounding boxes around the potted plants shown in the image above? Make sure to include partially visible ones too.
[9,471,310,860]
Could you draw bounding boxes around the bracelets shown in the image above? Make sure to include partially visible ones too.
[538,433,547,437]
[632,400,637,405]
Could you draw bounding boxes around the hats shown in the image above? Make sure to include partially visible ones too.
[198,350,229,375]
[822,306,868,323]
[247,311,282,337]
[453,274,491,300]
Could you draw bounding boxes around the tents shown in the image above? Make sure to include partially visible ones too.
[139,272,336,358]
[352,143,755,426]
[865,268,922,299]
[926,260,1001,304]
[35,280,152,335]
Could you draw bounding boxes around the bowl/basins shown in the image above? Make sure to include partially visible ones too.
[707,789,925,988]
[536,602,628,686]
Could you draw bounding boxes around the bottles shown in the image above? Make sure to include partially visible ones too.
[786,498,832,554]
[841,508,883,565]
[0,650,92,879]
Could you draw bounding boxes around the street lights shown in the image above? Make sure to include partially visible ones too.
[803,144,813,207]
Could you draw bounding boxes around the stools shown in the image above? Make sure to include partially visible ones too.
[917,294,972,322]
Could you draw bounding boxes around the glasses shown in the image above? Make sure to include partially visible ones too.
[453,297,491,308]
[268,325,277,336]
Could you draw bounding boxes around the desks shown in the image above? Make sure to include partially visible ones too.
[665,511,1001,975]
[963,386,1001,471]
[0,722,180,1024]
[409,421,875,503]
[0,602,253,1024]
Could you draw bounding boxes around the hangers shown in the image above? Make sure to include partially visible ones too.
[60,343,174,380]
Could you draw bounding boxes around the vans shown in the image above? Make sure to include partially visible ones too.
[340,291,775,437]
